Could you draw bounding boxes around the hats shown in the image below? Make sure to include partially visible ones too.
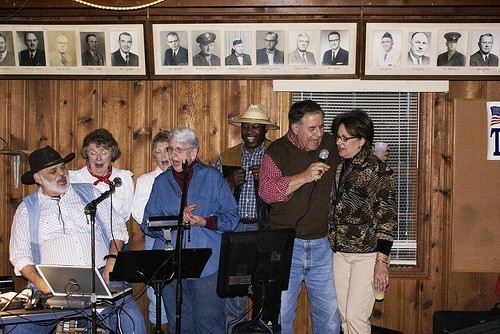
[444,32,461,40]
[196,32,216,44]
[22,146,75,185]
[382,32,392,40]
[229,104,280,130]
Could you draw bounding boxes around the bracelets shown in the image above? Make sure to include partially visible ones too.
[375,254,389,266]
[101,252,119,261]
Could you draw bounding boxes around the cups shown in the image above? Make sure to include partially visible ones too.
[371,280,385,300]
[375,142,388,164]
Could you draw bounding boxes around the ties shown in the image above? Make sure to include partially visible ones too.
[332,52,335,61]
[94,53,100,65]
[302,52,306,63]
[417,59,420,66]
[126,54,129,65]
[0,54,2,62]
[384,54,387,62]
[61,54,66,66]
[485,56,489,64]
[30,54,34,62]
[173,52,176,58]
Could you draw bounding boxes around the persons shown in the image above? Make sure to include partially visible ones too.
[193,33,221,66]
[6,146,146,334]
[49,33,74,66]
[321,32,348,66]
[130,130,179,334]
[224,39,252,66]
[214,102,278,333]
[288,32,316,65]
[406,32,434,68]
[63,128,135,225]
[141,127,239,334]
[19,32,45,66]
[438,31,467,66]
[81,33,104,67]
[111,32,140,66]
[0,33,15,66]
[257,32,284,65]
[471,31,499,67]
[378,33,402,67]
[257,100,392,334]
[326,108,394,334]
[162,31,189,66]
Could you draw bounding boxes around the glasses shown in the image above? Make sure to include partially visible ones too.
[169,147,194,154]
[329,39,340,42]
[57,42,67,45]
[168,40,179,43]
[25,39,37,42]
[337,136,357,141]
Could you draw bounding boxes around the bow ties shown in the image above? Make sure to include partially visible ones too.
[86,160,113,186]
[266,51,275,55]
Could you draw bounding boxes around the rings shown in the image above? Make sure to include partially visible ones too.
[318,169,323,175]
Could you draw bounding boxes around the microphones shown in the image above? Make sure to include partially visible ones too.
[315,148,330,182]
[110,177,122,187]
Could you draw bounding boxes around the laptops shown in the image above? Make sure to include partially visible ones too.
[34,265,132,300]
